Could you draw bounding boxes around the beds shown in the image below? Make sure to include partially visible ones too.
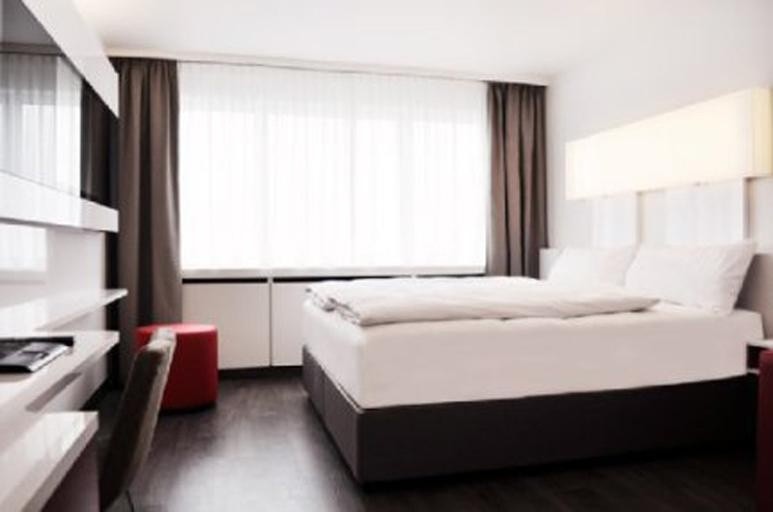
[301,247,772,489]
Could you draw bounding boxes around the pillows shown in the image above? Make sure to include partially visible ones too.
[546,233,755,315]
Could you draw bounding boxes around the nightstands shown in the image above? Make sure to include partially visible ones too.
[746,338,773,511]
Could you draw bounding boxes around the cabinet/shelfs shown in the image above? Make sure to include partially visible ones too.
[0,0,139,512]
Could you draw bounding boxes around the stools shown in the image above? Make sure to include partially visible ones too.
[135,323,221,414]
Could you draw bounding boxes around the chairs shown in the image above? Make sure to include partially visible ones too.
[99,327,182,512]
